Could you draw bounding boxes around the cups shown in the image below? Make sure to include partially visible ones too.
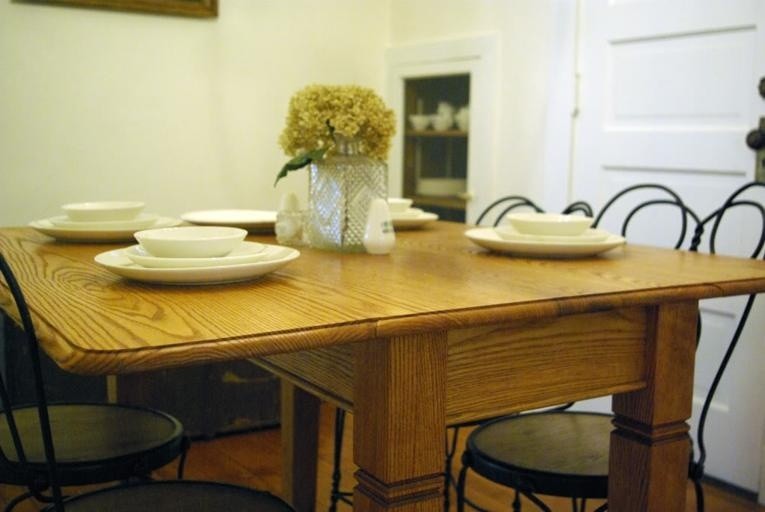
[408,104,470,133]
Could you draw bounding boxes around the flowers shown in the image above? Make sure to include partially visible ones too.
[273,82,397,188]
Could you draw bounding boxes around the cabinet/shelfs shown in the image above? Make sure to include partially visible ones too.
[403,73,469,222]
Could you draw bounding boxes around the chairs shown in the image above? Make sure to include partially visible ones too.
[40,480,296,512]
[0,251,191,512]
[329,196,592,511]
[456,182,764,512]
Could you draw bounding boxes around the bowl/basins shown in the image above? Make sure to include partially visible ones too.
[380,194,414,210]
[129,222,251,257]
[58,201,147,222]
[507,214,595,235]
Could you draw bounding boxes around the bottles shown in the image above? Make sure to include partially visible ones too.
[364,198,396,254]
[272,189,303,246]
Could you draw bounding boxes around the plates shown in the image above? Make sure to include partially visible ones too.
[93,244,298,285]
[462,226,627,259]
[25,216,184,243]
[413,176,467,203]
[180,209,277,231]
[390,208,440,230]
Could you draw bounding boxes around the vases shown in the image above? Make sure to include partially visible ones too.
[309,143,388,252]
